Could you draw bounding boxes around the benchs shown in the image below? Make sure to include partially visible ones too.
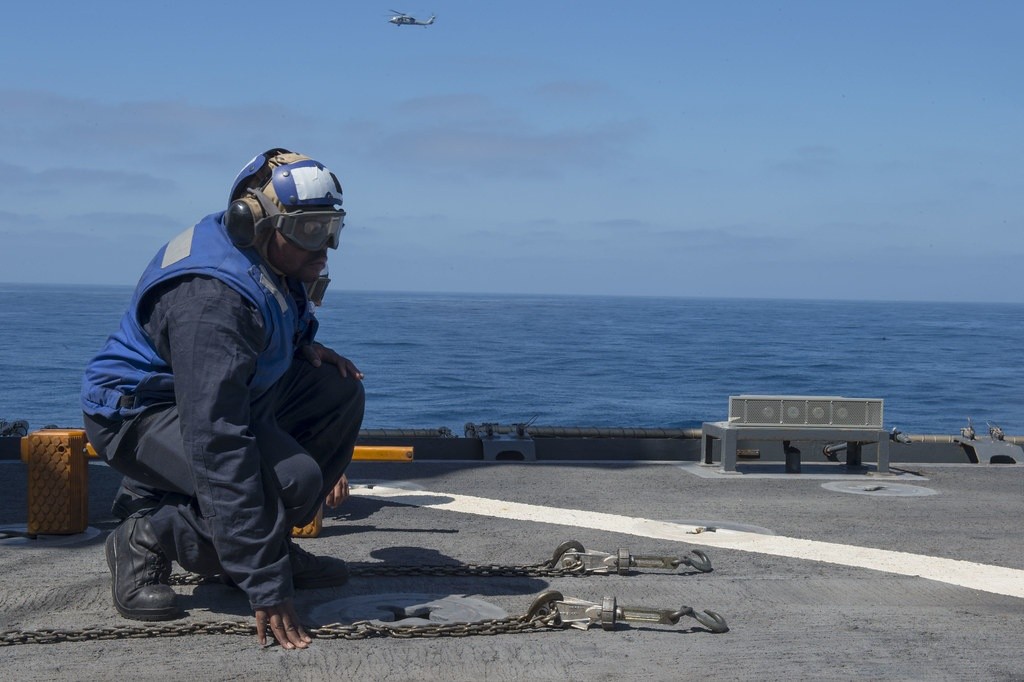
[696,395,897,477]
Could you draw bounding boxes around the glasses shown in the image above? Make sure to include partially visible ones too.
[270,211,347,251]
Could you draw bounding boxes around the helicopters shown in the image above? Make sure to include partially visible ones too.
[384,7,436,27]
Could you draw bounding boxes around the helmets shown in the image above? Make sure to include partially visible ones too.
[227,147,344,206]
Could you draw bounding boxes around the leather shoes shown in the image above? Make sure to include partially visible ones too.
[102,514,184,621]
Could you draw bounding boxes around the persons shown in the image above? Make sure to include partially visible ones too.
[80,147,365,650]
